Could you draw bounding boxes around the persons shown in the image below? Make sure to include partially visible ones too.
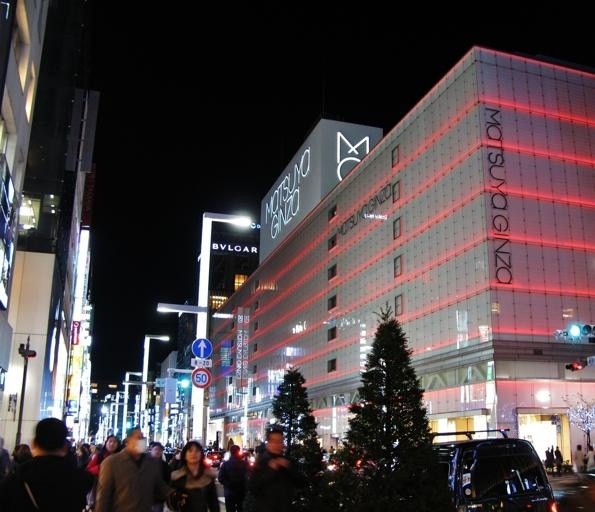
[543,448,554,471]
[571,445,583,480]
[585,446,595,473]
[554,446,564,476]
[1,416,295,512]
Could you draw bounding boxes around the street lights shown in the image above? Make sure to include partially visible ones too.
[101,211,251,442]
[330,393,345,434]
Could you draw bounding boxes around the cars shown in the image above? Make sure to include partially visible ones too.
[431,429,558,512]
[204,450,256,471]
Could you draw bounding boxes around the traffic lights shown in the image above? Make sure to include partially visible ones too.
[565,357,587,371]
[554,321,595,343]
